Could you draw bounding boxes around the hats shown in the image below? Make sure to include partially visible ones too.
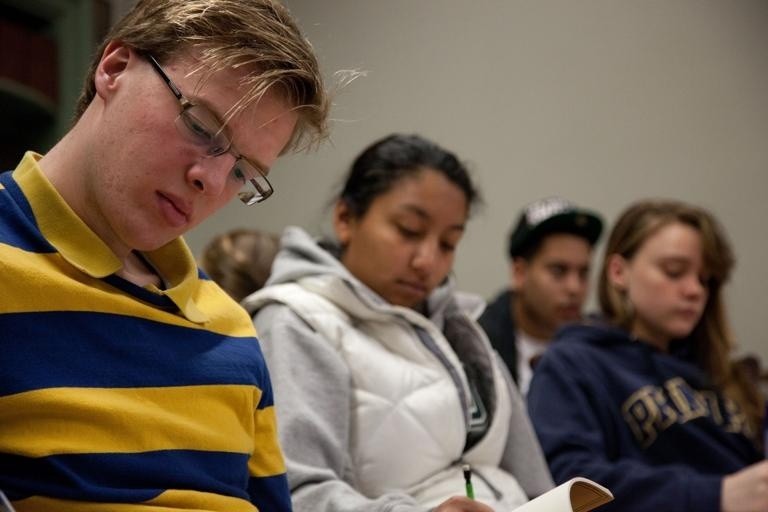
[509,199,605,260]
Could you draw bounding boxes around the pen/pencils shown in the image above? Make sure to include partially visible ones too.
[463,464,474,500]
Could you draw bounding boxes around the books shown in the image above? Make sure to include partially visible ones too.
[507,474,615,512]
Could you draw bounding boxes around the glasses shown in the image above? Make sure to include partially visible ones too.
[143,49,274,207]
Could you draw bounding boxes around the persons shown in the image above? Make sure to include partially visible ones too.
[1,0,369,512]
[477,195,604,401]
[239,128,556,512]
[199,227,281,302]
[523,198,767,512]
